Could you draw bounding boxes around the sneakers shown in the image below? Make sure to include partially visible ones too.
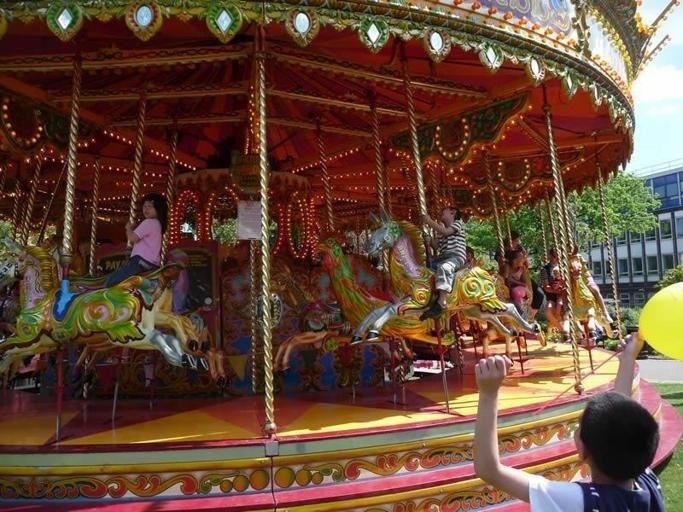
[601,312,613,323]
[562,332,571,343]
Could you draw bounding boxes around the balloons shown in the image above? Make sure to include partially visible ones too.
[638,282,683,361]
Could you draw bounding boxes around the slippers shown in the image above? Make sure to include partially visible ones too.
[420,306,441,321]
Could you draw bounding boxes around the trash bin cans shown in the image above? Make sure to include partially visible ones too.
[626,324,648,360]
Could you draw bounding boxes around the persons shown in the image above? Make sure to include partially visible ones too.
[473,332,665,512]
[494,232,544,322]
[423,207,467,318]
[105,193,167,290]
[540,243,614,343]
